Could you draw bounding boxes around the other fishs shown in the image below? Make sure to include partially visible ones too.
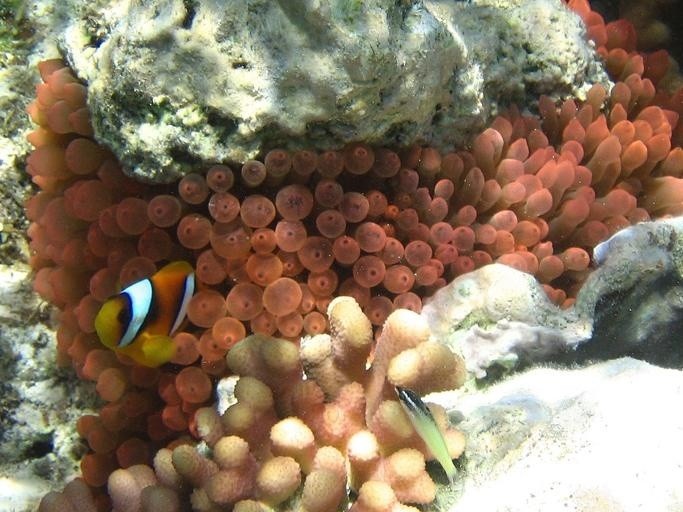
[393,383,460,485]
[94,260,198,370]
